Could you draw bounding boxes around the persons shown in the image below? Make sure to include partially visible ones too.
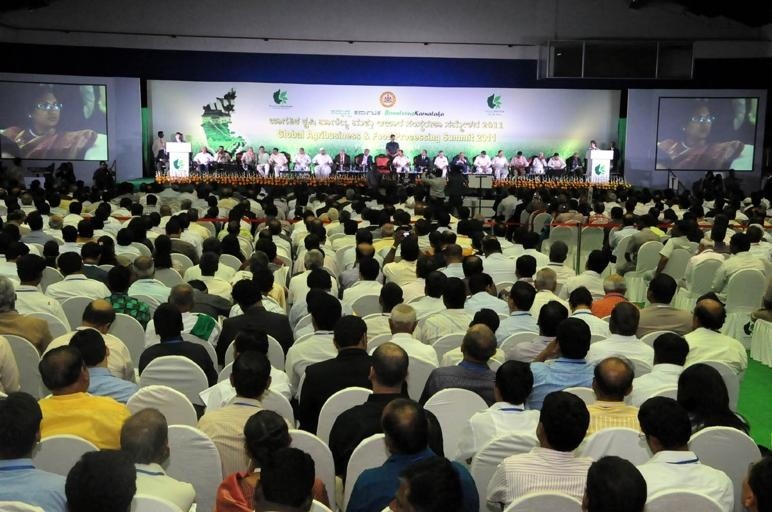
[655,99,716,169]
[2,85,62,159]
[149,131,619,178]
[0,161,771,511]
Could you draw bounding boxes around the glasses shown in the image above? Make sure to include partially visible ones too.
[693,113,718,123]
[34,102,63,112]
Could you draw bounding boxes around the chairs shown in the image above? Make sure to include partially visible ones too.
[1,149,772,512]
